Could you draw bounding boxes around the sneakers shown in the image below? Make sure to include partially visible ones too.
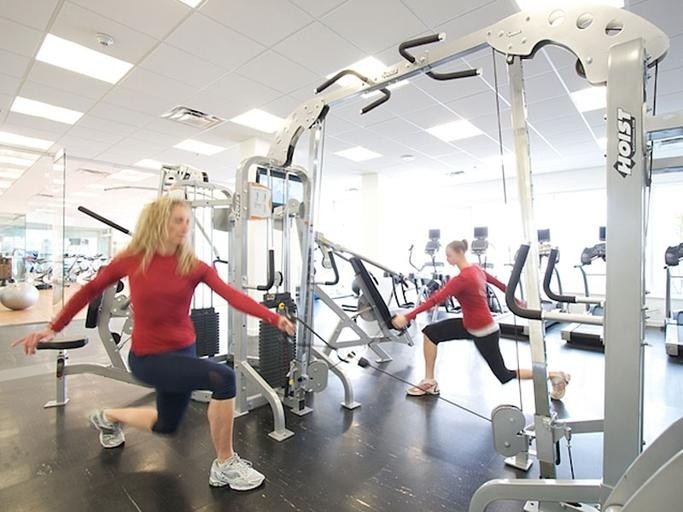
[550,372,571,400]
[88,409,125,448]
[407,380,440,395]
[209,452,265,491]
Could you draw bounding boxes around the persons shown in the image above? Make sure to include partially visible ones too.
[11,195,296,492]
[390,239,573,402]
